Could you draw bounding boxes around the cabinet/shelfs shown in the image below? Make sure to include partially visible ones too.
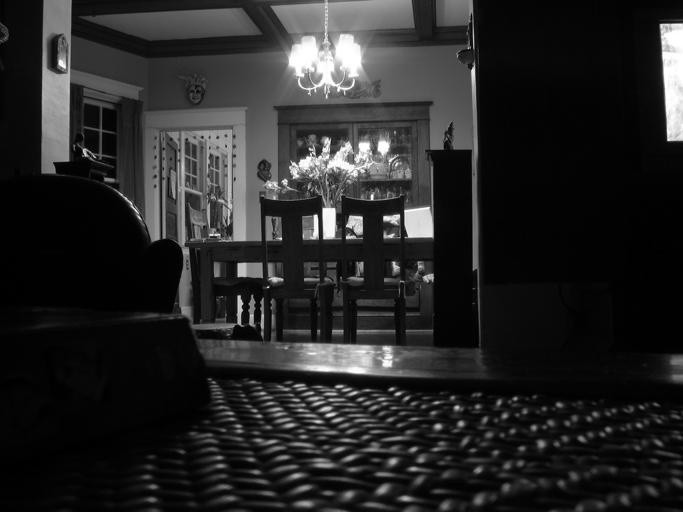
[277,105,432,211]
[424,142,478,345]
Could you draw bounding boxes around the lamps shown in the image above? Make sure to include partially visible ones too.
[284,3,361,96]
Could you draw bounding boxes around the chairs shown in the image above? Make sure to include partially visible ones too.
[340,194,409,348]
[185,201,263,328]
[260,192,335,337]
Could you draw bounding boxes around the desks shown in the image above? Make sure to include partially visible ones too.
[187,236,436,341]
[0,303,207,491]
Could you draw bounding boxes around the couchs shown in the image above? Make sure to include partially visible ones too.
[1,172,183,312]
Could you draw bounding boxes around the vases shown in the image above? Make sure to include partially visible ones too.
[313,205,335,237]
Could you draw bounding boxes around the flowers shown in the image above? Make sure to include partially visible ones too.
[261,136,392,203]
